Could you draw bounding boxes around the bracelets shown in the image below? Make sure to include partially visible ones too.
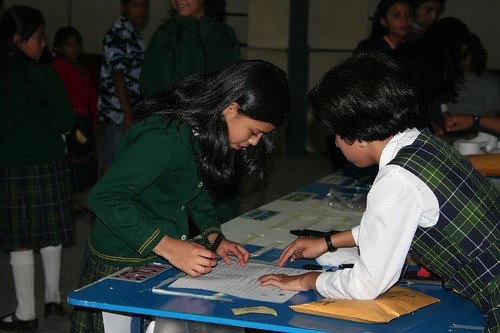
[473,112,480,130]
[324,230,336,252]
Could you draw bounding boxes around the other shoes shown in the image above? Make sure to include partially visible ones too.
[44,301,72,322]
[0,312,39,333]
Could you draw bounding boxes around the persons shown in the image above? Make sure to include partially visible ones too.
[140,0,241,106]
[257,53,500,333]
[443,111,500,134]
[458,32,500,111]
[404,0,446,41]
[97,0,150,179]
[354,0,414,68]
[49,26,98,214]
[68,58,292,333]
[386,17,473,135]
[1,3,77,333]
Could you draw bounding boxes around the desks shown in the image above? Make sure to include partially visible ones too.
[66,168,500,333]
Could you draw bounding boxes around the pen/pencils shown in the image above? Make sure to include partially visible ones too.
[303,264,339,270]
[211,234,225,252]
